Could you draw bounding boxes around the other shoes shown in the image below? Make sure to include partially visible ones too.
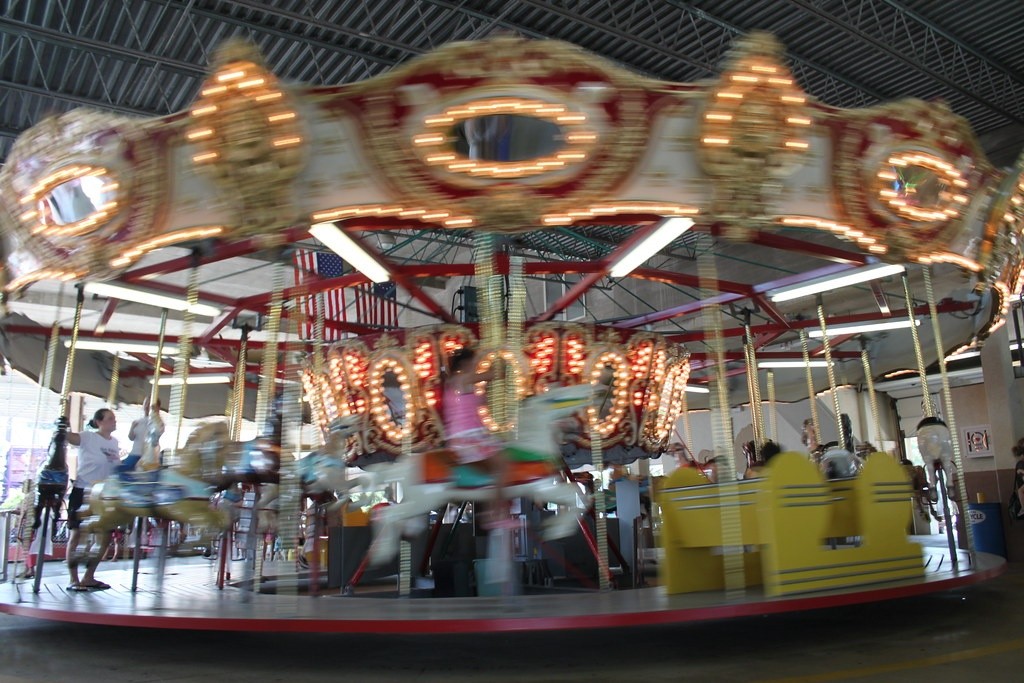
[15,572,35,579]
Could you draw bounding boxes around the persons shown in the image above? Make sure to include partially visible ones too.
[100,528,122,563]
[52,408,121,591]
[440,347,520,531]
[1012,437,1024,518]
[118,395,165,473]
[260,533,276,562]
[15,478,37,579]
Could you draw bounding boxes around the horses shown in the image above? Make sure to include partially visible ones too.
[916,397,956,502]
[359,382,611,568]
[64,412,361,569]
[604,457,730,502]
[32,415,70,539]
[819,449,864,479]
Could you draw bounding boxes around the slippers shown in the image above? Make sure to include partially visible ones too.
[82,581,111,589]
[66,584,90,592]
